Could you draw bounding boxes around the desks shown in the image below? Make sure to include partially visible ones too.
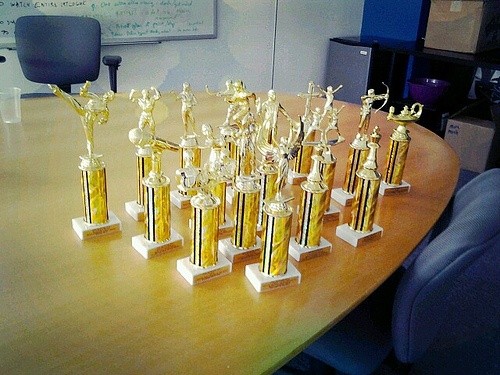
[0,88,459,375]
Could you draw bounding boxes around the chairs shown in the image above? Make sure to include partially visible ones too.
[280,167,500,375]
[14,15,121,98]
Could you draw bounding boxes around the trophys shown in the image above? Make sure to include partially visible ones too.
[48,78,424,294]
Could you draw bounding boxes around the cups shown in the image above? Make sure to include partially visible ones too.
[0,87,22,123]
